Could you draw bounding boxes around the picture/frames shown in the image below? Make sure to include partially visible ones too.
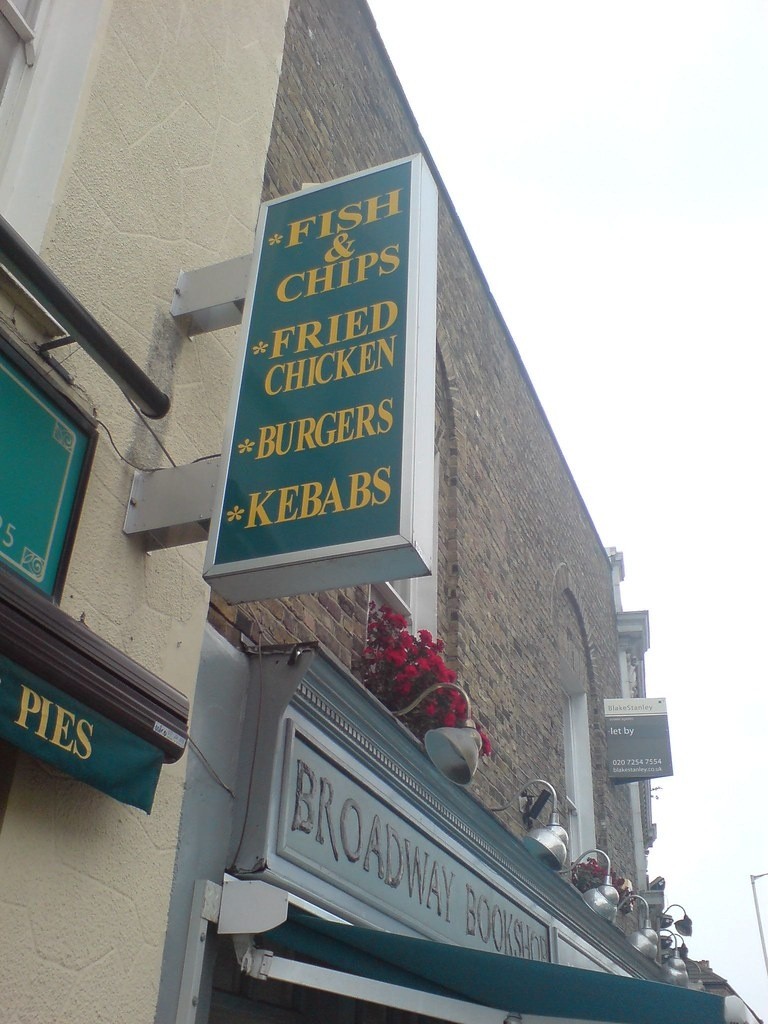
[0,335,99,607]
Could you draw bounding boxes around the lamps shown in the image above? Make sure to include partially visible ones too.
[660,905,693,936]
[490,778,569,871]
[657,929,686,977]
[686,958,704,990]
[560,848,620,923]
[391,682,482,787]
[618,894,658,960]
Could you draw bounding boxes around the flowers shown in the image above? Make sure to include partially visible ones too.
[571,857,634,913]
[362,599,492,758]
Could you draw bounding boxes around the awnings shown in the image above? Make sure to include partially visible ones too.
[234,910,751,1024]
